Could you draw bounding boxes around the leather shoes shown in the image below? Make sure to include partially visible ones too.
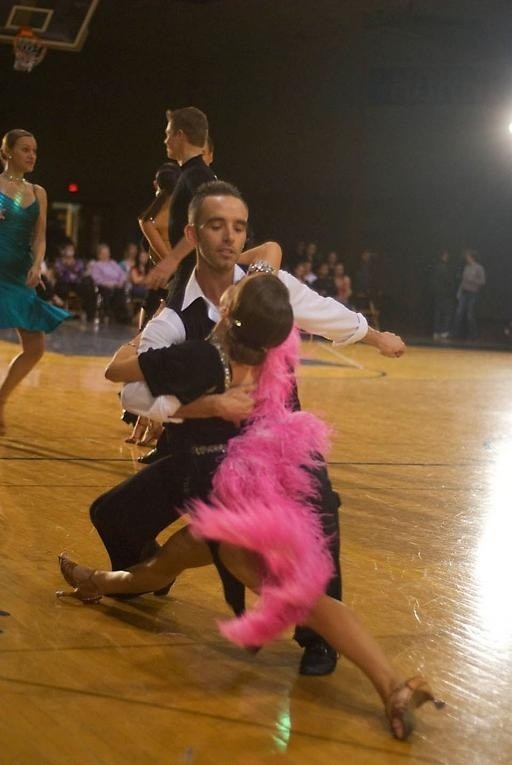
[301,643,336,676]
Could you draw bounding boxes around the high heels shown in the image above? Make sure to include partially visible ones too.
[122,411,167,463]
[56,551,103,603]
[388,675,444,740]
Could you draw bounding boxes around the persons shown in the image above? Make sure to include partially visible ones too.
[119,108,218,444]
[285,242,370,311]
[55,241,445,743]
[89,181,405,676]
[433,250,485,340]
[37,243,150,330]
[0,129,71,430]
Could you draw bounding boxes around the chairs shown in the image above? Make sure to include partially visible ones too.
[34,281,380,331]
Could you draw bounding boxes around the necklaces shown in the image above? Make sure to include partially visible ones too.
[3,170,25,184]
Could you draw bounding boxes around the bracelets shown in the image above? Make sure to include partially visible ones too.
[128,342,138,352]
